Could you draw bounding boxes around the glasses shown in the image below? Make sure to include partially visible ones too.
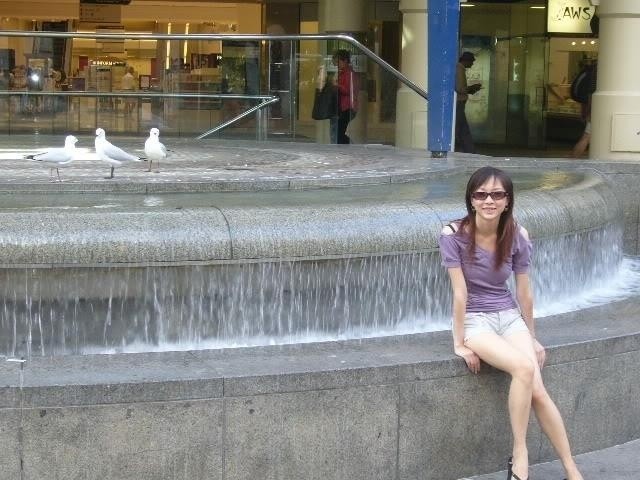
[473,191,507,200]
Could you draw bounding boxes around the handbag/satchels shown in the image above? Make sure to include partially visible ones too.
[570,72,591,104]
[313,85,337,120]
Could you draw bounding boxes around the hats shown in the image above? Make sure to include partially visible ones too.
[461,51,476,61]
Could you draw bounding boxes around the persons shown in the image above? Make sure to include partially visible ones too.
[438,165,587,480]
[122,66,140,123]
[456,49,483,154]
[570,57,596,158]
[324,48,359,144]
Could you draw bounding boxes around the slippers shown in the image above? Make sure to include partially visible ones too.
[507,457,529,479]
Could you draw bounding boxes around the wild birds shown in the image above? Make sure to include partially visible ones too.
[94,127,147,180]
[144,127,173,174]
[23,134,80,183]
